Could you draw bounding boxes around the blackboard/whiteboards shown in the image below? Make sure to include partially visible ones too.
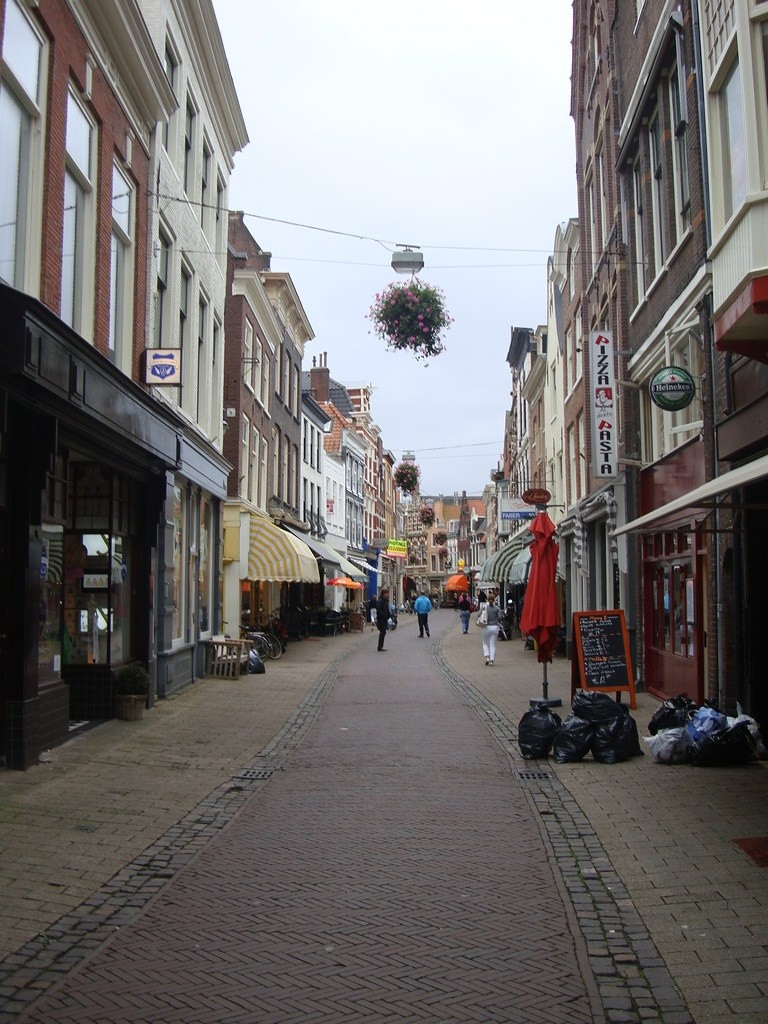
[573,610,635,694]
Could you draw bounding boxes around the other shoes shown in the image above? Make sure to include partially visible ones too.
[418,635,423,637]
[490,662,494,666]
[485,659,489,666]
[463,630,468,634]
[426,631,430,637]
[377,648,387,651]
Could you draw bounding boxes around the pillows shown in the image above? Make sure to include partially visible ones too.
[212,636,227,657]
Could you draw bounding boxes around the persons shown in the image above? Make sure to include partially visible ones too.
[428,588,442,611]
[414,590,432,638]
[478,589,493,609]
[454,592,471,636]
[376,589,391,652]
[325,605,341,632]
[477,594,502,666]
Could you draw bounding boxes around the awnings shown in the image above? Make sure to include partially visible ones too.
[509,535,560,585]
[445,574,468,591]
[613,454,768,536]
[482,540,522,583]
[249,516,320,583]
[283,523,367,582]
[348,555,386,574]
[403,576,417,592]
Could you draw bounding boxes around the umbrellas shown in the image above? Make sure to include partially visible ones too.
[326,577,363,609]
[519,502,560,683]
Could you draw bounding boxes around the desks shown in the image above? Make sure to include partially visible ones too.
[323,615,345,636]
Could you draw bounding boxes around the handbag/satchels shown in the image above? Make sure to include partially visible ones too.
[476,605,487,627]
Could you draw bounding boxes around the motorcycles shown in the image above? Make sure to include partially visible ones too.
[494,599,513,640]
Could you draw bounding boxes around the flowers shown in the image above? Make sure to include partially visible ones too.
[364,271,456,366]
[394,460,421,497]
[419,505,449,559]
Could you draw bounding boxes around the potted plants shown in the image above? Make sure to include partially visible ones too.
[114,665,150,721]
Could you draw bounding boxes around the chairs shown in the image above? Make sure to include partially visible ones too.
[349,613,364,632]
[205,637,254,681]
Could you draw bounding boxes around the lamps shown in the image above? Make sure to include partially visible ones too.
[390,244,425,274]
[402,450,416,462]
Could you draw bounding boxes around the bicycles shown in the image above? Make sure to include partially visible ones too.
[210,605,282,663]
